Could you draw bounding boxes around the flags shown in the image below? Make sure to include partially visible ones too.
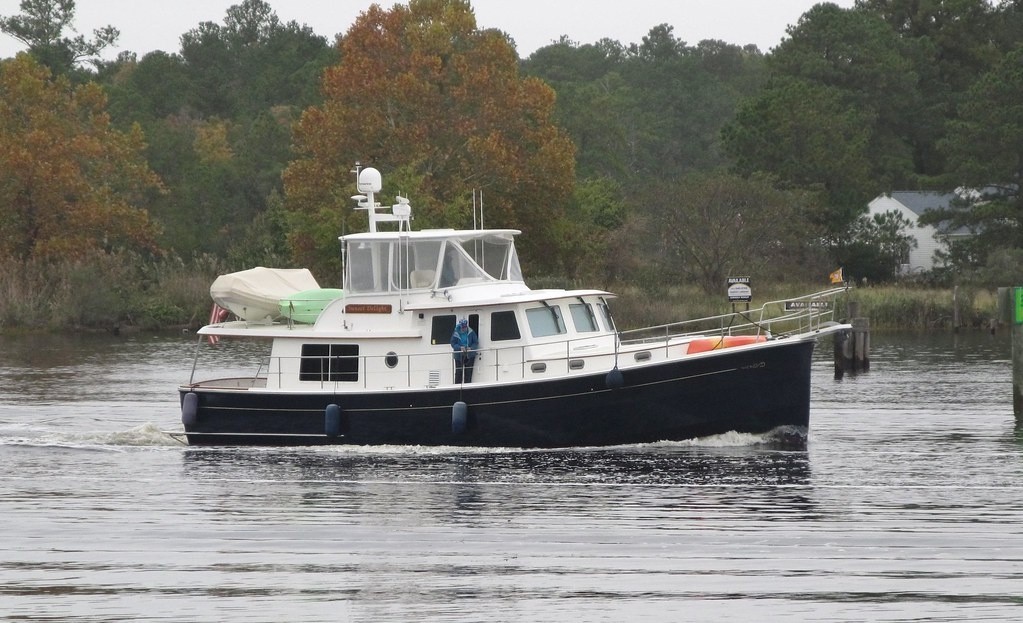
[829,267,842,284]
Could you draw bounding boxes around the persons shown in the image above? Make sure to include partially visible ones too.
[451,319,478,384]
[440,251,458,287]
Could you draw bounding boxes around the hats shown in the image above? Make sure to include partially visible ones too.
[459,320,467,328]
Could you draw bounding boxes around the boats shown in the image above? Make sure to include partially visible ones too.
[163,157,872,454]
